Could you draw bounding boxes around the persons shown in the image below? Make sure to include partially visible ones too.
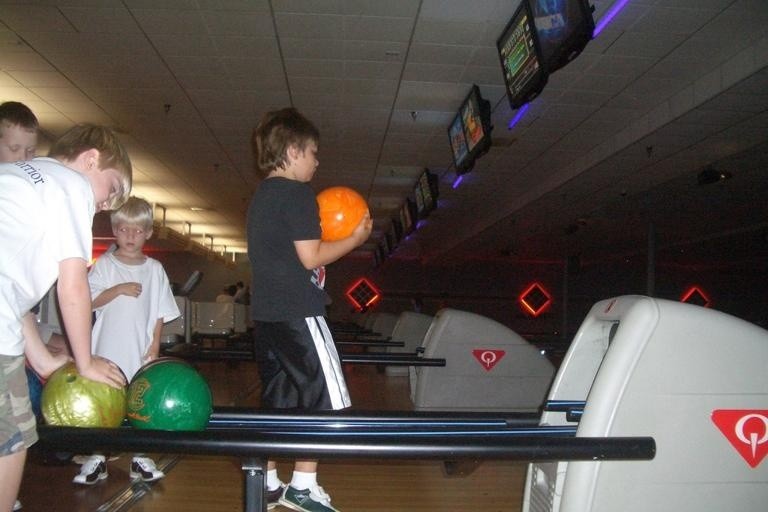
[439,295,448,308]
[415,297,422,313]
[73,197,181,485]
[0,102,132,512]
[248,107,374,512]
[216,282,251,305]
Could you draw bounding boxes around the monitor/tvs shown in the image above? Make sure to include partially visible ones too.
[447,111,475,176]
[496,0,548,111]
[374,169,439,268]
[524,0,595,76]
[458,85,492,160]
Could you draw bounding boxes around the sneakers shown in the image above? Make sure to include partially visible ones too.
[266,484,341,512]
[72,456,109,486]
[130,455,166,482]
